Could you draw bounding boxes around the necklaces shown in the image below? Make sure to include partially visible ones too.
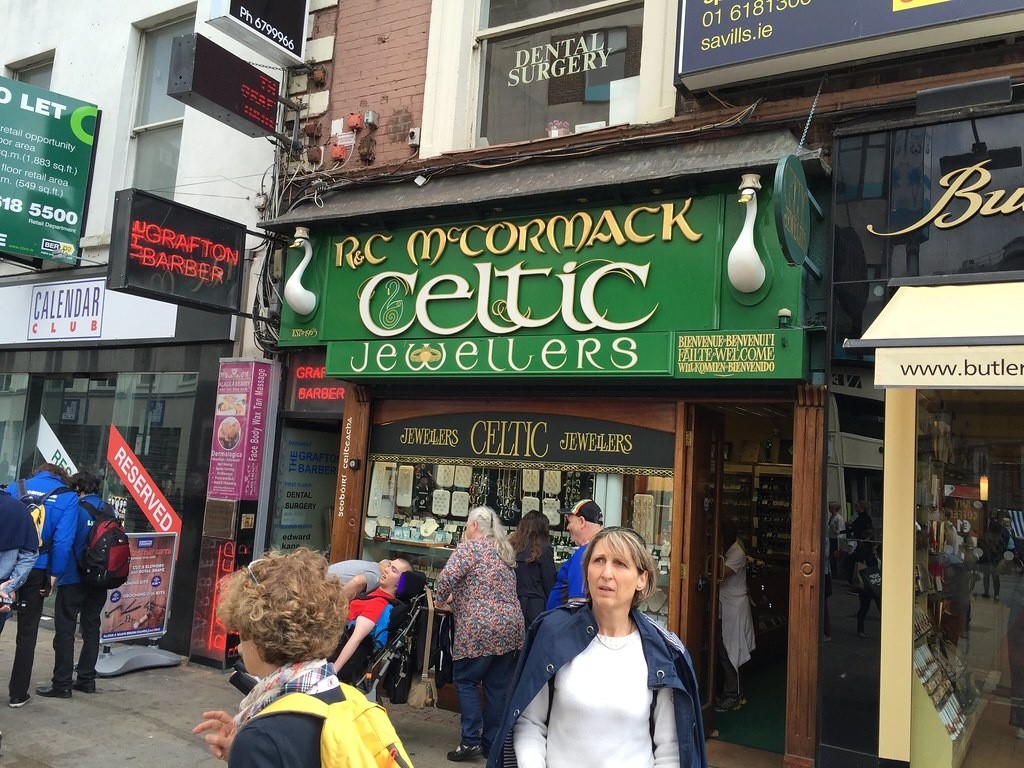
[588,611,635,651]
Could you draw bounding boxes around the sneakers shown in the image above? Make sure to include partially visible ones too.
[1015,727,1024,739]
[9,694,31,707]
[736,689,747,704]
[824,635,831,642]
[714,696,743,713]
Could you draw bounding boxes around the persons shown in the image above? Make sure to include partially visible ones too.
[313,554,394,606]
[827,492,882,640]
[433,506,527,762]
[947,504,1024,744]
[545,498,605,609]
[35,470,132,698]
[0,464,79,709]
[0,481,40,741]
[191,546,415,767]
[314,557,414,676]
[709,514,757,714]
[506,509,560,647]
[484,525,710,768]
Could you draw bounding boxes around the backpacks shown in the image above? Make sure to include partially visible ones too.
[17,479,76,554]
[245,681,415,768]
[72,501,131,589]
[849,562,876,595]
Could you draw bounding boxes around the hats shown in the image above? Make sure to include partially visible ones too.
[557,499,603,525]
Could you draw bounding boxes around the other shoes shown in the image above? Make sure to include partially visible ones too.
[447,743,483,761]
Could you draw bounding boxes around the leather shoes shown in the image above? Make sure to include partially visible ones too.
[36,686,72,698]
[72,680,95,693]
[857,632,871,638]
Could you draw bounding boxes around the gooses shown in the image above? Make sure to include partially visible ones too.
[727,188,766,292]
[285,238,316,315]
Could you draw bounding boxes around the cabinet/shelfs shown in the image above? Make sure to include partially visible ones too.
[708,462,793,574]
[910,600,977,768]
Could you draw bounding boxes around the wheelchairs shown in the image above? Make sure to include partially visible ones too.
[226,570,434,709]
